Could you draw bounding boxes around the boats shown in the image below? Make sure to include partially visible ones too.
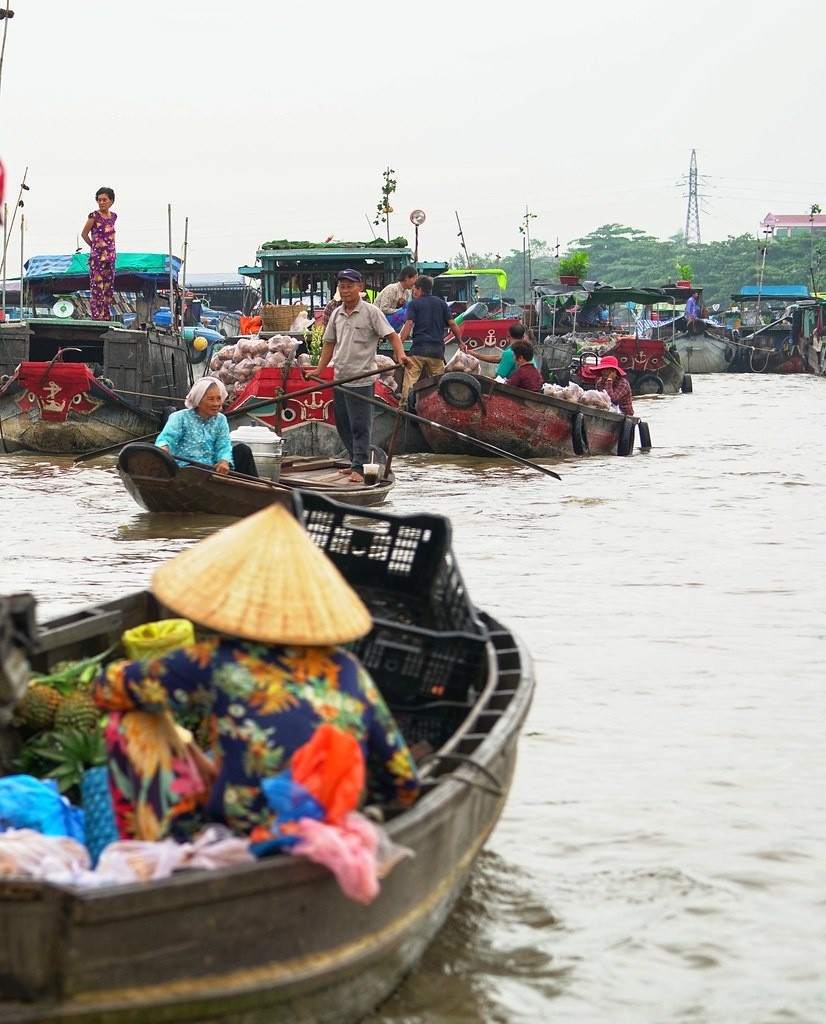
[115,441,399,516]
[0,203,826,476]
[1,486,536,1023]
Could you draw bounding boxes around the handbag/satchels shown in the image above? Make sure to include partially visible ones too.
[0,826,92,883]
[0,773,87,843]
[95,827,256,887]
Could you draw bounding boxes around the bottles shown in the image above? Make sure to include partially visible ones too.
[152,307,172,326]
[9,306,32,319]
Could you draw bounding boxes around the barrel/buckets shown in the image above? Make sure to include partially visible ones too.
[314,307,325,331]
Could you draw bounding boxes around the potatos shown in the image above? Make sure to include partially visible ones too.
[545,382,610,410]
[207,336,311,383]
[446,351,480,373]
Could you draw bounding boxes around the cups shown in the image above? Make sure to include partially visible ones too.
[363,464,380,486]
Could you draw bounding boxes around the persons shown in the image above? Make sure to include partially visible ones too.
[373,266,418,343]
[589,356,634,415]
[575,305,605,326]
[97,505,418,855]
[685,291,698,321]
[536,293,552,328]
[81,187,117,321]
[301,269,414,482]
[505,342,545,394]
[155,377,259,479]
[322,287,365,330]
[467,324,537,380]
[393,276,468,411]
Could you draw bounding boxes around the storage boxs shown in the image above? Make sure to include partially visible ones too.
[389,681,475,752]
[289,487,490,709]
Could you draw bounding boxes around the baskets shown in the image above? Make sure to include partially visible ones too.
[259,301,308,331]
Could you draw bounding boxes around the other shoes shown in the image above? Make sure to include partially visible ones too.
[398,399,408,406]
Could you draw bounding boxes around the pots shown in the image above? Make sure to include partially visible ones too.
[229,420,287,483]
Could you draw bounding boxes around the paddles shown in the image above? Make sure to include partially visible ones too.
[382,395,405,483]
[74,361,404,464]
[167,450,297,493]
[301,368,562,480]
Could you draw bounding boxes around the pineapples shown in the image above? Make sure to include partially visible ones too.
[10,656,110,793]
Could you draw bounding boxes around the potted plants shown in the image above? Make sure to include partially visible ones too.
[676,263,696,287]
[554,250,592,285]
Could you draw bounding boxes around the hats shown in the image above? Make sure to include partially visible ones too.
[150,499,373,645]
[589,356,628,377]
[336,268,362,283]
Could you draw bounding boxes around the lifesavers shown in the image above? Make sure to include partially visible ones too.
[638,421,652,448]
[618,420,634,459]
[681,373,692,393]
[571,411,589,456]
[635,374,664,395]
[438,371,481,409]
